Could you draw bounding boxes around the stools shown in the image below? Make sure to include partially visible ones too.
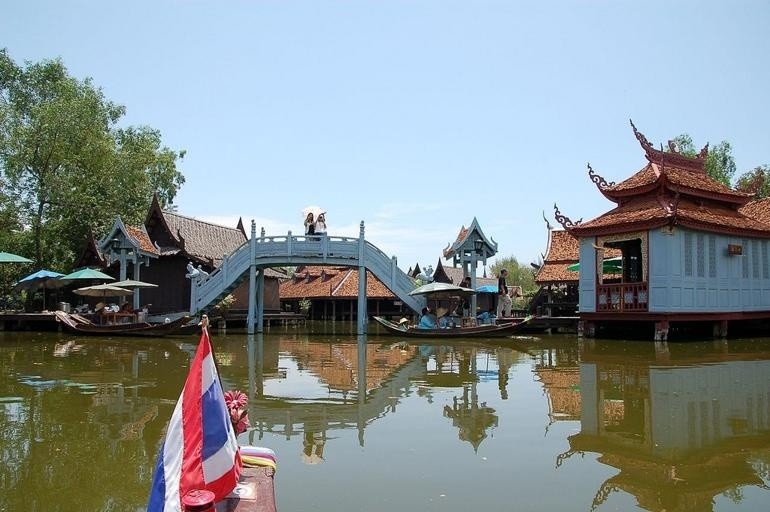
[460,316,476,328]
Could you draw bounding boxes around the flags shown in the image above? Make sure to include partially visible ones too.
[145,319,243,511]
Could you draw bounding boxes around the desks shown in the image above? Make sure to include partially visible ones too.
[100,313,135,324]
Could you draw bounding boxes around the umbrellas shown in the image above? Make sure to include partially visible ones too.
[56,268,115,305]
[406,282,463,296]
[565,256,635,276]
[106,275,160,291]
[69,281,136,307]
[11,267,65,312]
[422,287,473,297]
[0,250,37,311]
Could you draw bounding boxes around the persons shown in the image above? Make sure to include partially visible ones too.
[418,306,436,329]
[455,276,472,317]
[304,212,314,239]
[435,306,450,328]
[315,213,328,241]
[496,269,512,317]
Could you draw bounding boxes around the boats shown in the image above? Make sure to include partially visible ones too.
[372,314,536,339]
[53,310,192,336]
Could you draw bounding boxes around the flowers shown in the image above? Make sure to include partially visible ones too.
[225,389,250,438]
